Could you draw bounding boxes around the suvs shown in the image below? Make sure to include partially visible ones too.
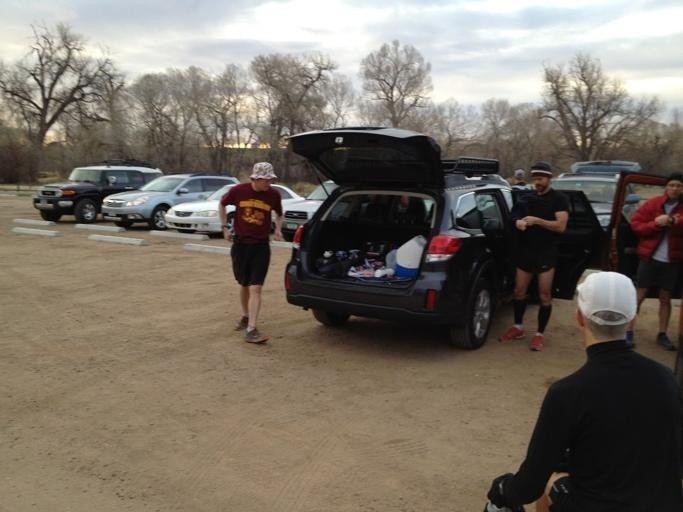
[99,172,240,230]
[31,158,164,226]
[545,156,644,237]
[282,125,610,350]
[279,178,339,242]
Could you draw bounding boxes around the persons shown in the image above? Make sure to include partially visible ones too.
[512,169,534,200]
[218,162,284,343]
[483,269,683,512]
[625,171,682,352]
[498,163,564,351]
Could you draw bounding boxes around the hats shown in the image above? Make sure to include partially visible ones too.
[514,162,552,180]
[577,271,637,325]
[250,162,277,179]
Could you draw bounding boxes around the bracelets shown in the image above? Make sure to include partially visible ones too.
[220,224,230,230]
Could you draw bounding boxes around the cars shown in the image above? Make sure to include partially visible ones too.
[163,182,304,240]
[607,169,682,300]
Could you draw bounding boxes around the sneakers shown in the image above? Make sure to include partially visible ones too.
[625,331,635,347]
[501,325,544,351]
[657,332,674,349]
[235,316,269,343]
